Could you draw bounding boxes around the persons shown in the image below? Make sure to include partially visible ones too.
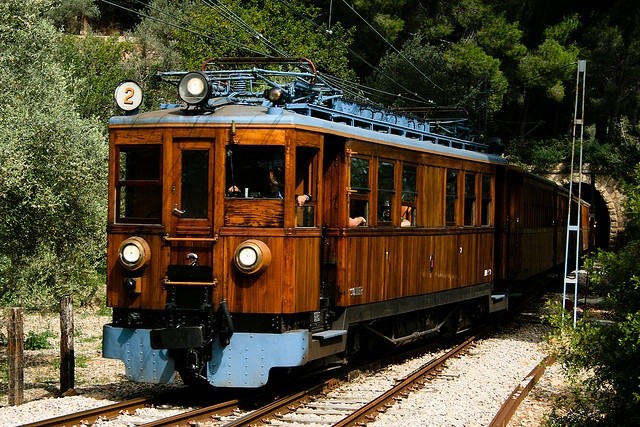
[348,216,366,228]
[228,177,311,207]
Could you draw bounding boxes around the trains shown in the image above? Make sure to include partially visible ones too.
[102,55,599,387]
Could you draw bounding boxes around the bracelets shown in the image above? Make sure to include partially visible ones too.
[306,194,310,201]
